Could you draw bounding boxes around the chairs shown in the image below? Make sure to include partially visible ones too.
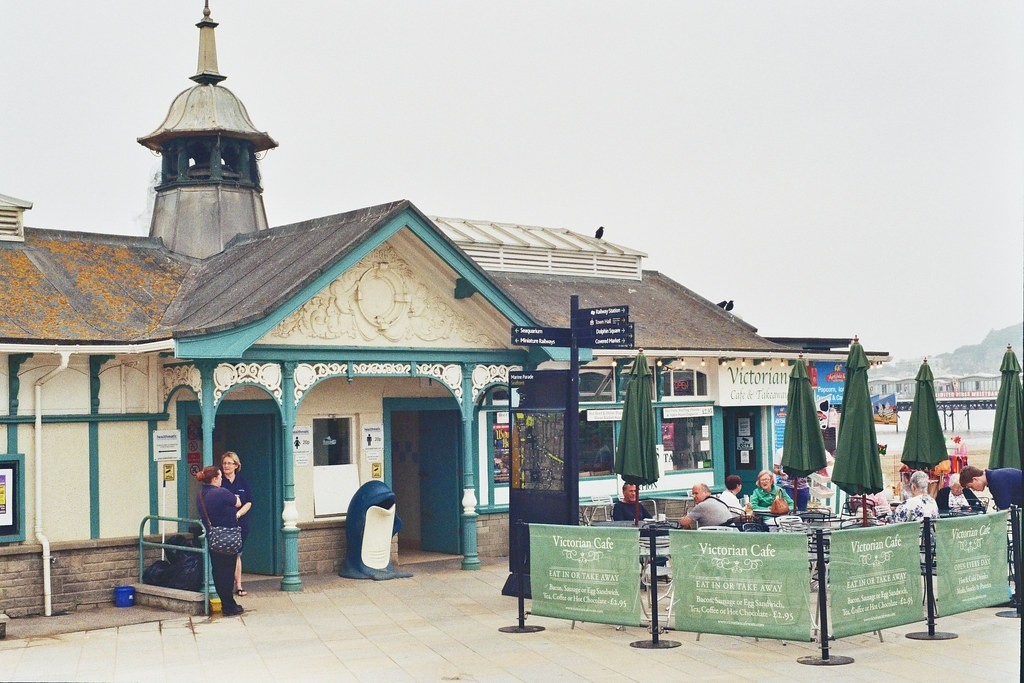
[575,498,989,644]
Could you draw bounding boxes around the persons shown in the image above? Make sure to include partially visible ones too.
[885,470,940,524]
[935,473,986,515]
[220,451,253,596]
[849,492,893,516]
[680,482,736,528]
[718,475,751,515]
[774,447,811,511]
[196,466,244,617]
[959,466,1024,510]
[750,470,798,511]
[613,483,653,521]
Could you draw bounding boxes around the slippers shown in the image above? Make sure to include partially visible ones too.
[235,590,247,596]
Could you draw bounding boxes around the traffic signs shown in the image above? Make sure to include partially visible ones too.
[510,305,636,350]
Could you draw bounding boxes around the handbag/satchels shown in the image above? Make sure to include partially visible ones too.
[771,488,789,514]
[209,526,242,554]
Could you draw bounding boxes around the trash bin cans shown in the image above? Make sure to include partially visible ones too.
[337,480,413,581]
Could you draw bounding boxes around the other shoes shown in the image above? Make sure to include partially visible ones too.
[1009,598,1017,608]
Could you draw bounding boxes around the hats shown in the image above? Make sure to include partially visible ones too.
[911,471,929,490]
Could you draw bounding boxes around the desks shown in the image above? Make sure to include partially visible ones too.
[591,521,683,591]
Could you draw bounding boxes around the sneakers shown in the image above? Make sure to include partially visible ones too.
[223,605,244,616]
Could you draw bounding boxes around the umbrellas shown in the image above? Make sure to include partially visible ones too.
[784,334,1024,526]
[612,346,660,526]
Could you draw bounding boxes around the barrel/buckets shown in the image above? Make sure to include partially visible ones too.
[114,586,135,607]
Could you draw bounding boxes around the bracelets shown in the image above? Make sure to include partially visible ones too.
[237,512,240,519]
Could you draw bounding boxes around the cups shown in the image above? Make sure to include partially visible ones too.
[658,513,666,522]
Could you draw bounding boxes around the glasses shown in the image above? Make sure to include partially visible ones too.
[223,461,237,466]
[760,479,771,482]
